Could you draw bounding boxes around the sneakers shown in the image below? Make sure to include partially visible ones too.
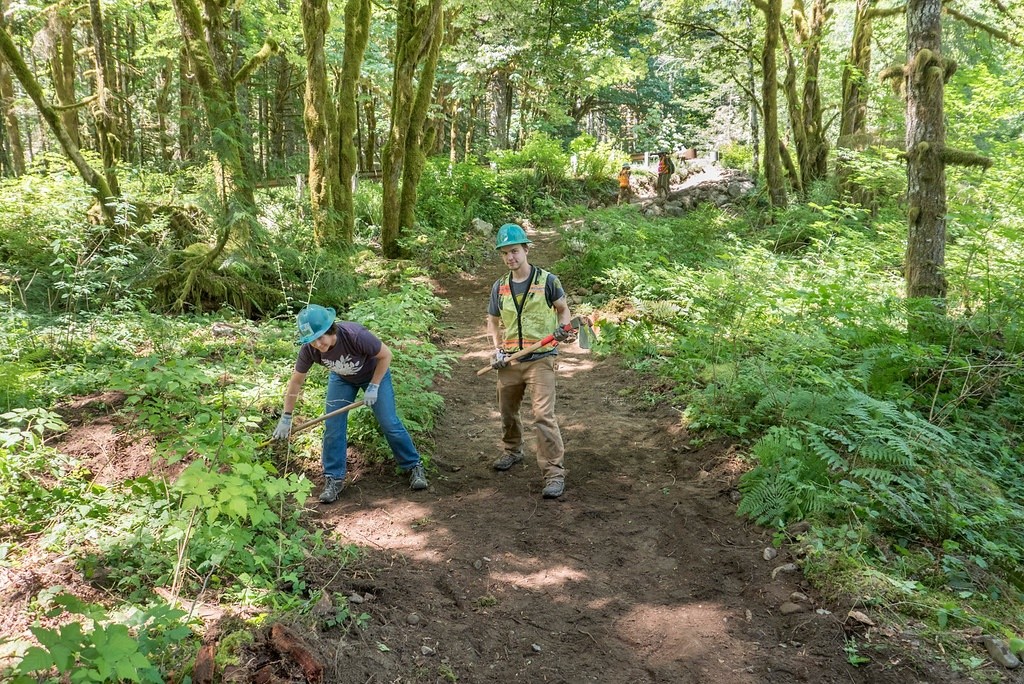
[319,479,344,501]
[407,461,428,489]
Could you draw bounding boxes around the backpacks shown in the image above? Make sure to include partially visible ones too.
[665,158,675,174]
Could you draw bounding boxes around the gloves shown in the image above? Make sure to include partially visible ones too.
[490,351,511,370]
[363,383,379,406]
[273,415,293,440]
[553,323,569,342]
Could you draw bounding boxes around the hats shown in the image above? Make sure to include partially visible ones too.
[623,163,630,167]
[660,147,668,153]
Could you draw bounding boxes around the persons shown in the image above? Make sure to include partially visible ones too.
[657,148,675,199]
[486,223,571,498]
[616,162,632,207]
[271,304,428,504]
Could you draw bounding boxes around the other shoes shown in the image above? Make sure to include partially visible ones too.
[542,480,564,498]
[493,453,520,469]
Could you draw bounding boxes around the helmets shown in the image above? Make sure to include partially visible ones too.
[295,304,336,344]
[494,223,532,250]
